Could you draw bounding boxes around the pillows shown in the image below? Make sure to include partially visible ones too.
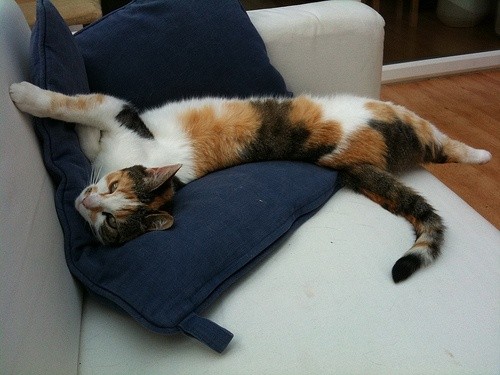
[23,0,347,355]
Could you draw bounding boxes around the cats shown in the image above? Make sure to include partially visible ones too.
[8,80,492,284]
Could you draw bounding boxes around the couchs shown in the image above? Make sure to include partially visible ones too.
[0,0,500,375]
